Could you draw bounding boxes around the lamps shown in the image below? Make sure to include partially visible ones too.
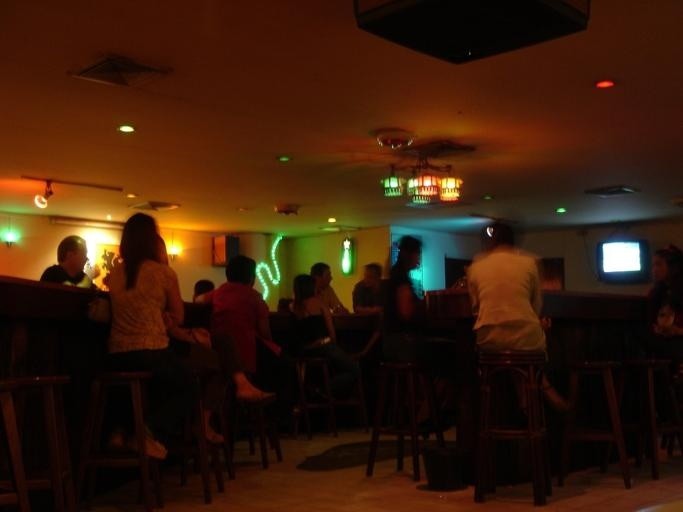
[30,179,56,210]
[366,135,479,211]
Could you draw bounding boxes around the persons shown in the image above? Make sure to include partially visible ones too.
[107,214,282,466]
[385,237,455,434]
[40,236,99,290]
[467,224,571,420]
[642,244,682,456]
[289,264,388,397]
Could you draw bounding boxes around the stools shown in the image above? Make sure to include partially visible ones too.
[0,345,682,512]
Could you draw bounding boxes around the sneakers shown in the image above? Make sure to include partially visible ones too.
[112,429,170,459]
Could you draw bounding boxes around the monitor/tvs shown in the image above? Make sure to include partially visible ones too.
[597,239,651,284]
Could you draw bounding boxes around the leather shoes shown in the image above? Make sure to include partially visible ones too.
[199,432,226,445]
[237,389,277,401]
